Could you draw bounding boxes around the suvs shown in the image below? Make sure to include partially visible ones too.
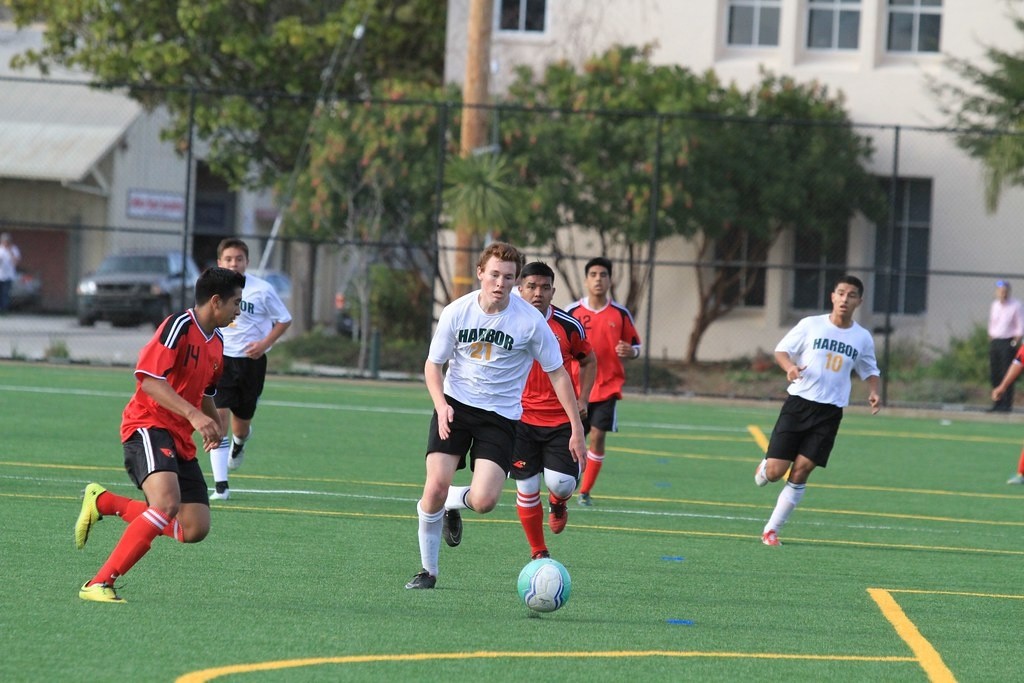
[76,249,202,330]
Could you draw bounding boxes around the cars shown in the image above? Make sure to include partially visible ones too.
[245,269,293,313]
[10,266,39,306]
[335,261,352,336]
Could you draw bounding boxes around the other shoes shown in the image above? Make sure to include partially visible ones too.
[988,406,1012,412]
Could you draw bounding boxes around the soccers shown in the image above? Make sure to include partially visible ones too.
[516,557,573,614]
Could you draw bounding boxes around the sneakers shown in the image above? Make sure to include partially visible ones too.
[209,488,230,500]
[1007,473,1024,485]
[75,482,107,551]
[761,528,783,546]
[755,458,768,487]
[443,509,463,547]
[531,550,549,560]
[549,493,568,534]
[406,568,436,589]
[227,425,253,470]
[78,579,129,603]
[578,493,594,506]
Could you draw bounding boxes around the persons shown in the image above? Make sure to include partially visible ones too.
[563,257,642,511]
[505,262,598,561]
[207,237,292,501]
[404,243,588,590]
[754,275,881,549]
[990,339,1024,485]
[985,280,1024,415]
[74,267,246,605]
[0,231,21,319]
